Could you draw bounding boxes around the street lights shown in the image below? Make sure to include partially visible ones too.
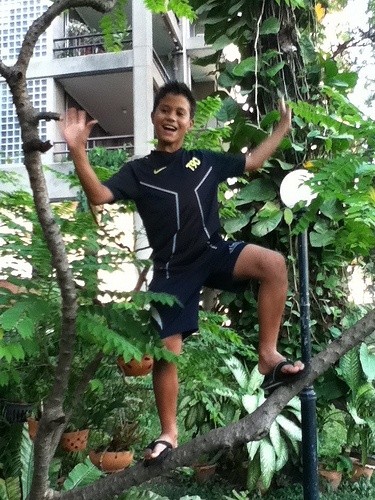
[278,168,324,499]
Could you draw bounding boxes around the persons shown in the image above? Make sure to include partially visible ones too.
[56,80,308,465]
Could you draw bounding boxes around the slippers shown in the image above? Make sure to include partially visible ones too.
[259,359,311,388]
[143,438,172,465]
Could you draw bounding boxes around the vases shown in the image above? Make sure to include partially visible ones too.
[319,468,344,490]
[116,354,153,377]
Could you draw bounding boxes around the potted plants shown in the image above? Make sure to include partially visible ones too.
[91,420,142,472]
[4,386,39,423]
[62,393,127,452]
[350,423,375,483]
